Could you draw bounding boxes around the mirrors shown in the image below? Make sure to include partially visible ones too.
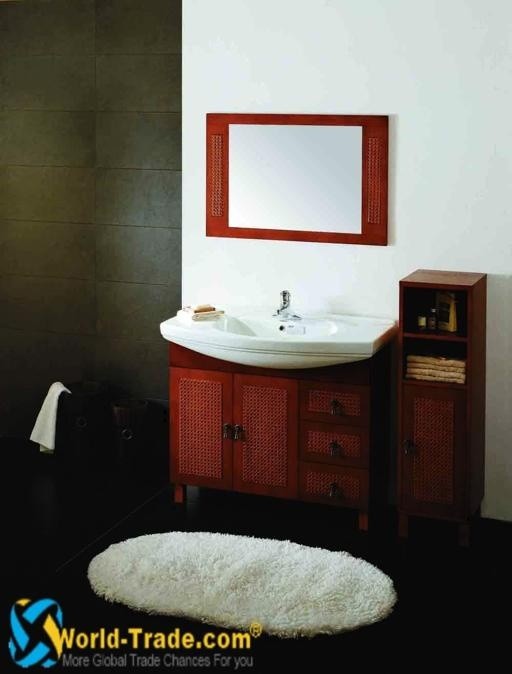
[206,114,388,246]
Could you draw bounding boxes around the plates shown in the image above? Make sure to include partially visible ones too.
[178,309,225,321]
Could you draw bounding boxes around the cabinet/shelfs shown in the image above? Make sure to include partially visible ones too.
[395,270,488,549]
[170,367,391,531]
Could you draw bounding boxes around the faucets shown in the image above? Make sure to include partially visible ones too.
[272,289,301,323]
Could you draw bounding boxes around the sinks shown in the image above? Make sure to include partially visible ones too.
[159,307,397,371]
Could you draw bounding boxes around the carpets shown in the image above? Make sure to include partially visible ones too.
[88,531,398,639]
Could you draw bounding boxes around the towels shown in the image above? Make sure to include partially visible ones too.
[29,381,72,455]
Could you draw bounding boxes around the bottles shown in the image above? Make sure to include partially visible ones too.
[417,306,439,332]
